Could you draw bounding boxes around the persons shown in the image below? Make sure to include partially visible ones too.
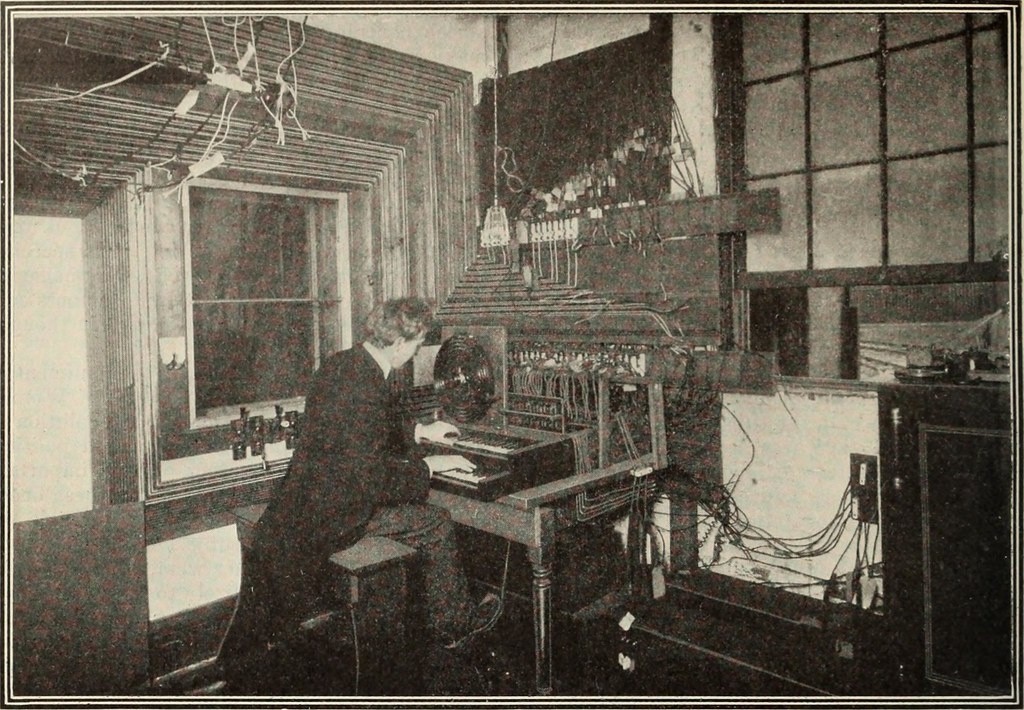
[224,297,505,696]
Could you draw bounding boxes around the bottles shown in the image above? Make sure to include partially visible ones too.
[233,422,247,459]
[251,418,264,455]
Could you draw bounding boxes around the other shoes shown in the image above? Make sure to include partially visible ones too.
[440,598,504,649]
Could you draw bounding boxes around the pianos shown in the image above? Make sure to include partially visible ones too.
[391,330,674,697]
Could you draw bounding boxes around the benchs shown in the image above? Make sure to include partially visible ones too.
[216,501,417,698]
